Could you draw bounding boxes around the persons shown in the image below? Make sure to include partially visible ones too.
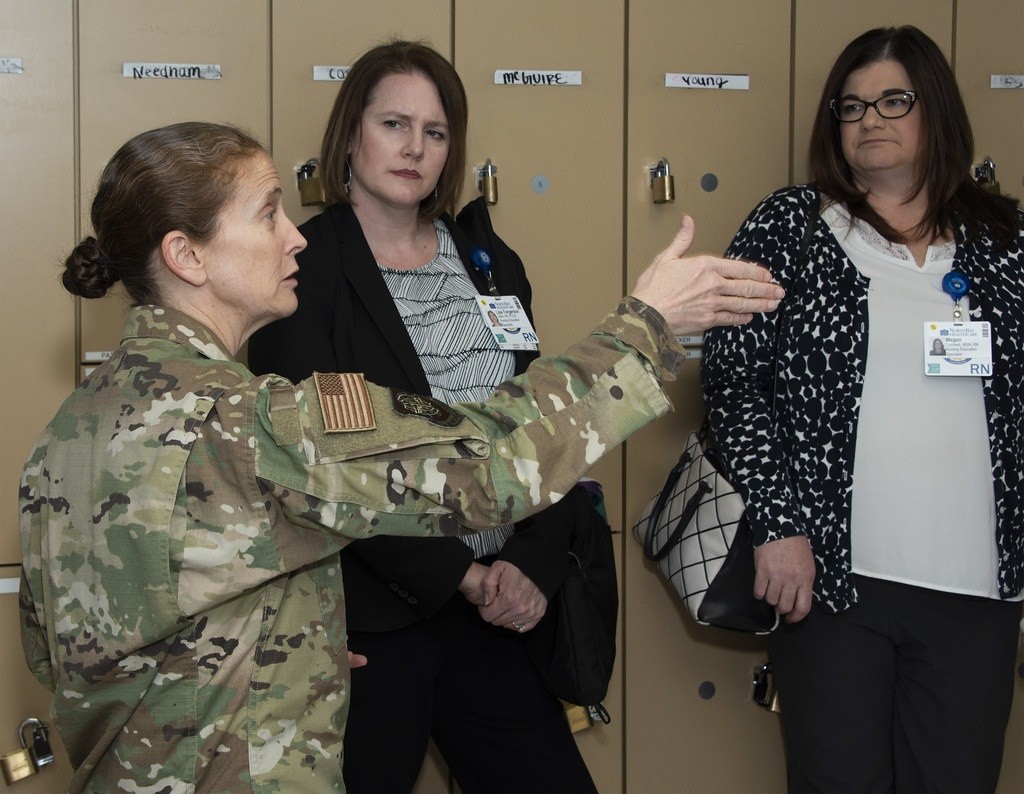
[244,41,599,794]
[700,23,1024,794]
[488,311,502,326]
[930,338,946,356]
[18,122,784,794]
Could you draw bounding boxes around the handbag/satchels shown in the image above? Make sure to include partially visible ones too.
[538,480,618,708]
[631,431,780,634]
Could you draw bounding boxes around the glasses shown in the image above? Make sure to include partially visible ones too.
[829,91,918,123]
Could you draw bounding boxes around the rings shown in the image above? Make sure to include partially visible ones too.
[512,622,525,631]
